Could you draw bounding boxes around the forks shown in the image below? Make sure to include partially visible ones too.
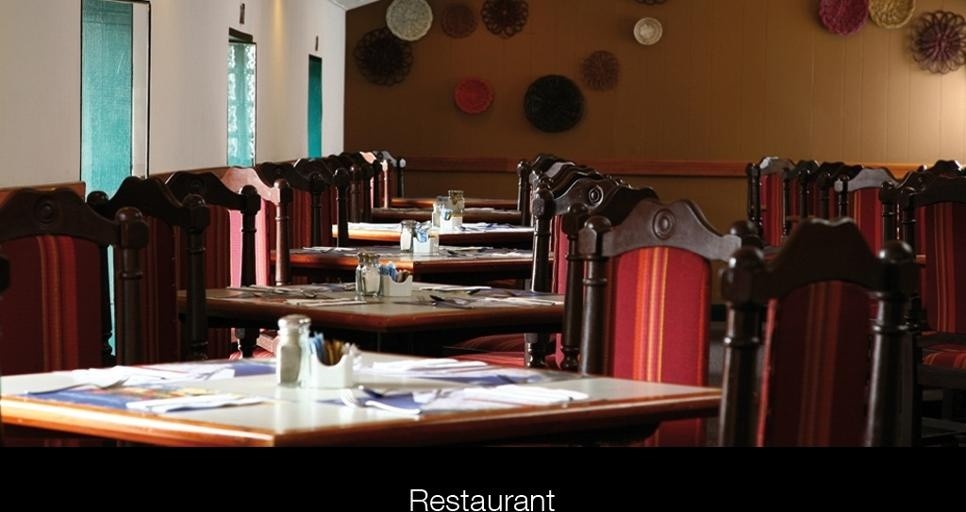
[414,295,475,309]
[24,378,134,395]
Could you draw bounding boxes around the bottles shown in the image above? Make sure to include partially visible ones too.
[275,314,312,389]
[355,251,382,298]
[400,220,417,253]
[447,190,464,214]
[431,195,453,230]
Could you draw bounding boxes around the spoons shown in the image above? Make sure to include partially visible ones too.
[428,294,480,303]
